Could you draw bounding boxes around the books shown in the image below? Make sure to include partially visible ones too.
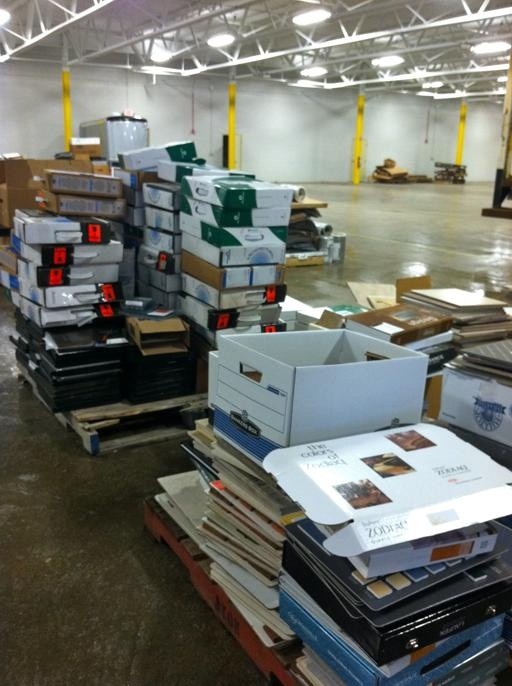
[345,287,512,387]
[180,417,511,685]
